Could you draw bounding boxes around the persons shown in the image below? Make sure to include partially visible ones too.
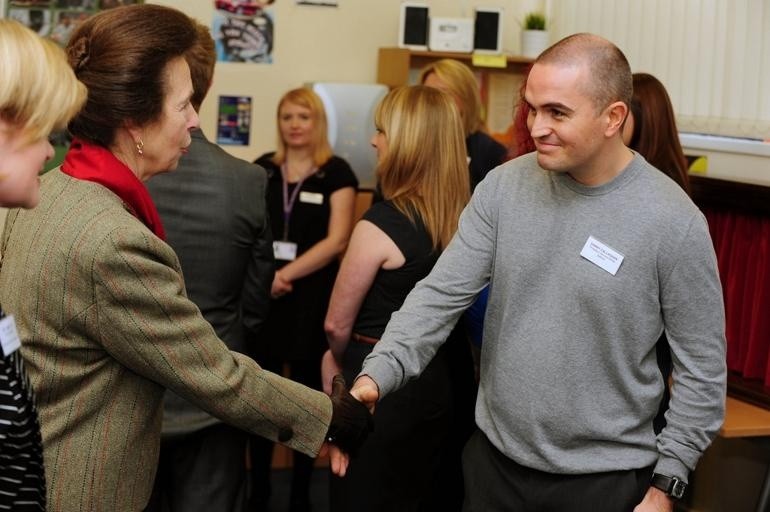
[0,20,88,511]
[0,3,371,511]
[621,72,690,194]
[319,34,728,511]
[139,19,511,511]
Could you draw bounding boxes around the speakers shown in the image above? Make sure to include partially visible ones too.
[474,10,503,53]
[398,2,430,51]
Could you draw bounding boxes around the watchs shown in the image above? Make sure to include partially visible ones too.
[651,472,686,499]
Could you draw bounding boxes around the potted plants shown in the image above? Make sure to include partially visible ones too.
[521,12,548,59]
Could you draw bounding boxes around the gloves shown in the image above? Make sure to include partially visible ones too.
[325,374,375,458]
